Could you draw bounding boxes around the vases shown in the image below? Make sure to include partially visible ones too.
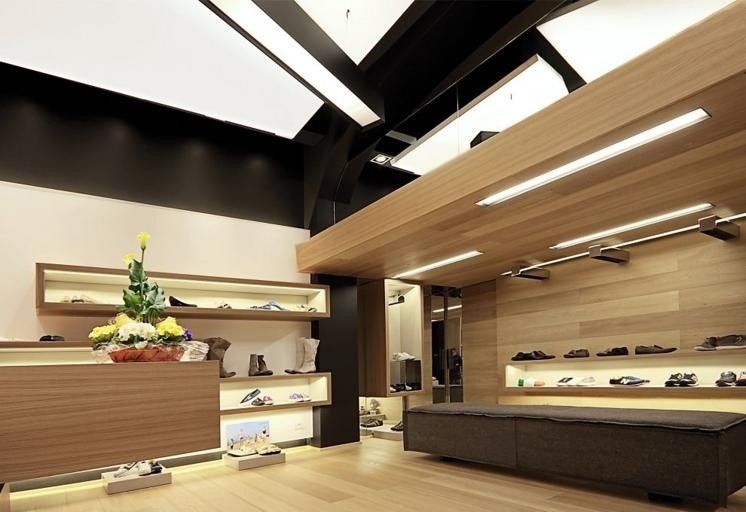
[104,342,186,364]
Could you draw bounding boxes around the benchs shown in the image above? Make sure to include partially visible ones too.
[516,405,746,511]
[401,401,517,468]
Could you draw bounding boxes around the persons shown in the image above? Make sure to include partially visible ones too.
[449,348,463,385]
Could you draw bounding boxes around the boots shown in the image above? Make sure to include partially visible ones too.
[285,337,320,374]
[204,337,235,378]
[249,354,274,376]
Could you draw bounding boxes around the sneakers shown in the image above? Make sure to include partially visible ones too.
[693,334,746,350]
[226,431,282,456]
[112,459,162,480]
[665,373,699,385]
[391,421,403,431]
[610,376,649,388]
[392,353,415,362]
[289,393,312,403]
[240,389,272,407]
[361,418,382,427]
[716,371,746,386]
[390,382,418,393]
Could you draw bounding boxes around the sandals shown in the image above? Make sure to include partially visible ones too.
[250,300,316,312]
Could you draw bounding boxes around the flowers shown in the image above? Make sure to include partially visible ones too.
[84,229,193,352]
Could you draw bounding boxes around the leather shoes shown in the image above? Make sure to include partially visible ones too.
[169,295,196,307]
[597,347,628,356]
[511,350,555,360]
[635,344,677,355]
[564,349,589,357]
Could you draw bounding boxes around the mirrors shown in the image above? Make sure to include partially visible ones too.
[431,296,461,387]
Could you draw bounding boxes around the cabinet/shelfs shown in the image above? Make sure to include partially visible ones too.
[391,360,420,385]
[505,344,746,400]
[34,261,331,322]
[219,371,333,416]
